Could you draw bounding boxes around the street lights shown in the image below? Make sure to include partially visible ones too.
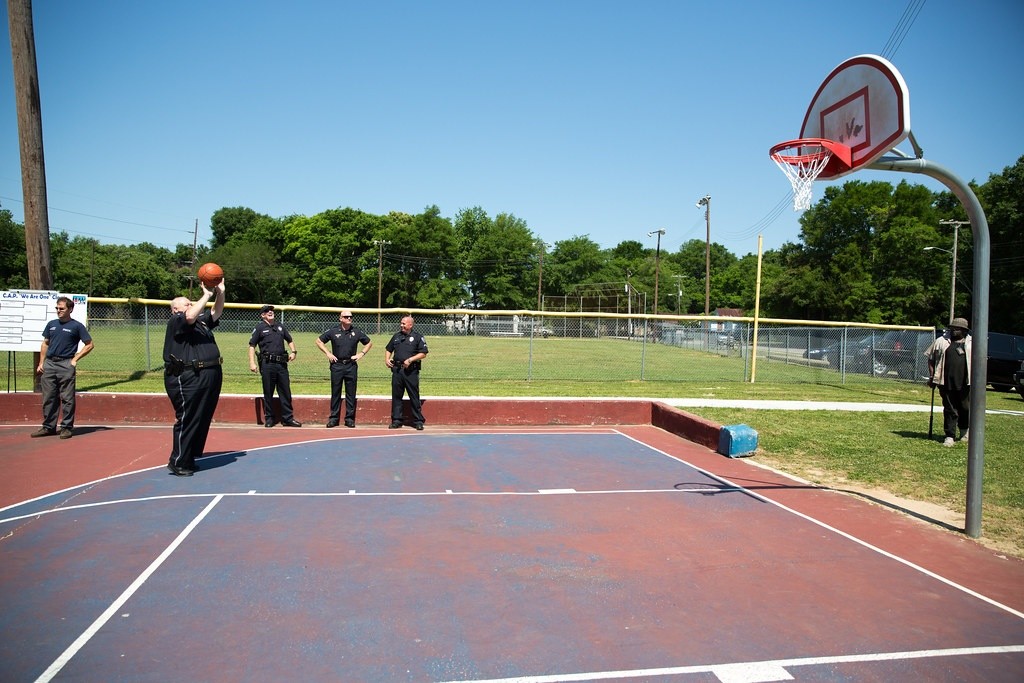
[373,239,391,334]
[924,219,971,326]
[647,228,666,343]
[667,275,687,315]
[696,195,712,352]
[531,242,552,337]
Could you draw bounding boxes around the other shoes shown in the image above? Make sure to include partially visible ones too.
[960,429,969,442]
[345,422,356,428]
[167,461,201,477]
[943,438,954,448]
[265,418,274,427]
[281,417,302,427]
[416,422,423,430]
[389,423,402,429]
[327,421,340,428]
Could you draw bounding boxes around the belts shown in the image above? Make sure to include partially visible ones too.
[183,357,224,368]
[47,356,72,362]
[338,359,351,364]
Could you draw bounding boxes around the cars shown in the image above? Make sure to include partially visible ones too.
[662,328,694,342]
[854,335,884,373]
[893,329,945,379]
[704,329,735,346]
[803,343,830,362]
[869,329,902,375]
[518,317,554,338]
[826,336,867,367]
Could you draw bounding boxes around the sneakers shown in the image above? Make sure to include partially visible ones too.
[60,427,75,438]
[31,426,58,437]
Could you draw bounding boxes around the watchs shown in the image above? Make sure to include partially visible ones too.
[407,358,412,363]
[292,351,297,354]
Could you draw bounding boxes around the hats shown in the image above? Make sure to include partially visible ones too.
[260,305,275,314]
[948,318,971,334]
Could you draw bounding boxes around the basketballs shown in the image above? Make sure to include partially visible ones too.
[198,263,224,288]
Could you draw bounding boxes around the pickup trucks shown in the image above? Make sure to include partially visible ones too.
[969,330,1024,398]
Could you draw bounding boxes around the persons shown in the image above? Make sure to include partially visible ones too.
[621,322,653,338]
[727,334,735,343]
[315,310,372,428]
[248,306,302,428]
[431,318,464,332]
[163,277,223,477]
[923,318,972,448]
[533,325,553,338]
[594,322,607,337]
[385,316,428,430]
[31,297,94,438]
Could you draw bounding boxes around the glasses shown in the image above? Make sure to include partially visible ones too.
[265,308,274,312]
[55,306,66,310]
[342,316,353,319]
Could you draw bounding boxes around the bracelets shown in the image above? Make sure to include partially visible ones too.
[361,350,365,355]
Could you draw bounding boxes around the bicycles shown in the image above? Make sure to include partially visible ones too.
[715,335,740,351]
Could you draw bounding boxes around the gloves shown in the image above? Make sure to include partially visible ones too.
[927,376,936,389]
